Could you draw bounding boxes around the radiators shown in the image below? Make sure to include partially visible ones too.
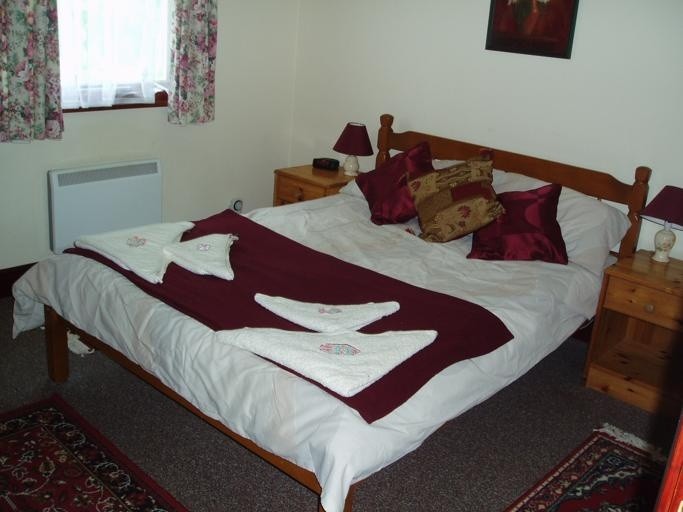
[45,156,164,257]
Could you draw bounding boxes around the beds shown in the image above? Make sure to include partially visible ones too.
[11,112,653,512]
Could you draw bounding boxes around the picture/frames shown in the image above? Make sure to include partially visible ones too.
[483,0,581,61]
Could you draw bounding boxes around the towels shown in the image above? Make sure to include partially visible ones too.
[73,221,195,285]
[213,325,439,399]
[252,291,401,333]
[163,233,239,282]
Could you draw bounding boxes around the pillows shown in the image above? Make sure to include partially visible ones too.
[403,151,508,243]
[354,141,442,225]
[493,173,633,279]
[465,181,568,264]
[338,148,507,203]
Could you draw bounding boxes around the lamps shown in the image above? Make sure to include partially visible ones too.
[331,120,374,177]
[638,182,683,264]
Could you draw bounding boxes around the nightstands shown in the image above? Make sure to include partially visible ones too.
[271,162,367,210]
[580,249,683,421]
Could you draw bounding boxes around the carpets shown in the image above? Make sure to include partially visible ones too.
[498,418,672,511]
[0,391,194,512]
